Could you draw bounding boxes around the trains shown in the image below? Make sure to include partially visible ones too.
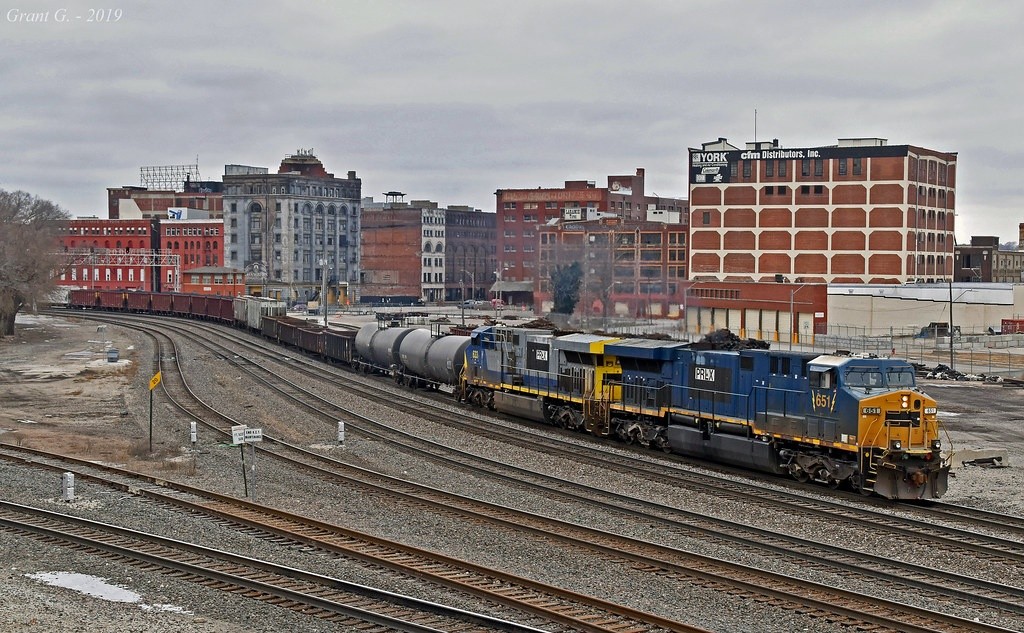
[263,312,950,501]
[68,288,288,333]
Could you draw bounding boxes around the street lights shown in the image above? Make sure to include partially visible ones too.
[946,278,954,370]
[325,267,333,327]
[458,266,510,324]
